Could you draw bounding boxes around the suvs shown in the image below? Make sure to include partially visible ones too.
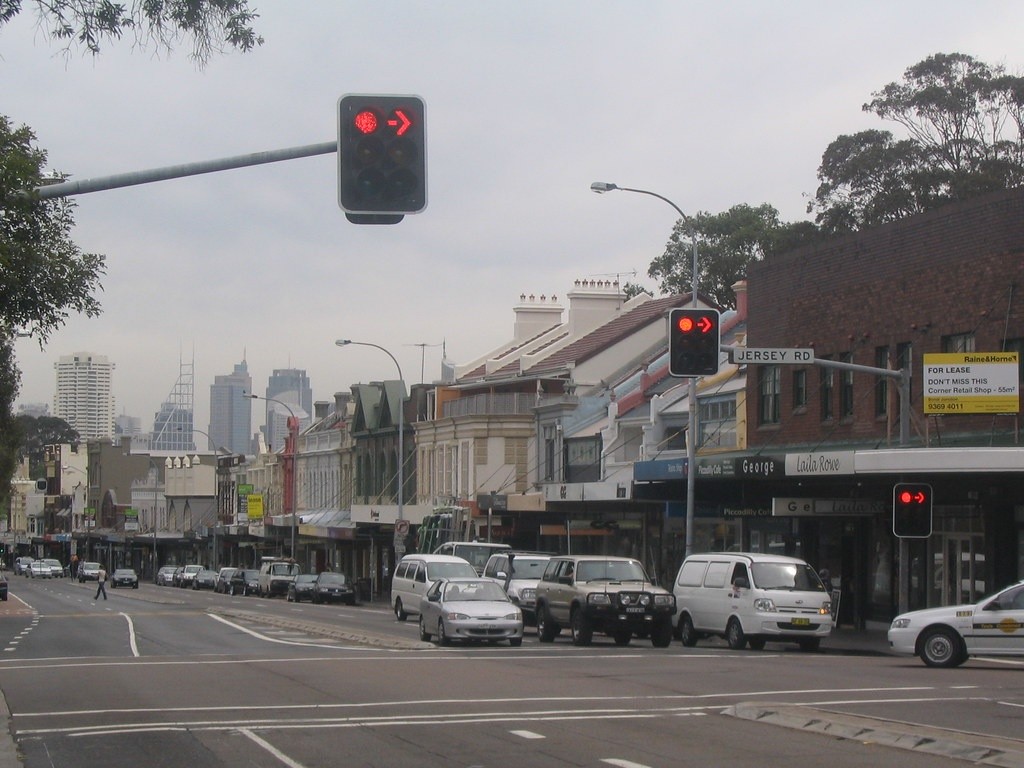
[482,551,563,635]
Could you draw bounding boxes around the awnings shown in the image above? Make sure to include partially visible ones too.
[57,508,72,518]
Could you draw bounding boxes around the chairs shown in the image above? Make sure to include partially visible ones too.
[452,585,459,593]
[475,588,483,596]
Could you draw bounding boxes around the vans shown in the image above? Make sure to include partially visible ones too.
[391,555,480,621]
[670,552,833,651]
[436,541,512,577]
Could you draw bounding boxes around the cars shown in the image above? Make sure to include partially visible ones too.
[535,556,678,648]
[78,561,104,582]
[418,578,524,646]
[0,556,66,601]
[887,581,1024,669]
[110,569,139,589]
[156,555,357,607]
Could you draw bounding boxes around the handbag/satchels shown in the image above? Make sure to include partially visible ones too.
[106,574,108,581]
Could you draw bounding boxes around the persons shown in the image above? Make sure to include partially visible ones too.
[94,564,109,600]
[569,564,590,580]
[69,554,85,583]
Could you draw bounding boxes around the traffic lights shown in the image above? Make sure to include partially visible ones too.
[669,307,719,377]
[892,483,934,538]
[336,94,427,215]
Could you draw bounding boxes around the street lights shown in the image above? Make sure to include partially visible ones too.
[123,452,159,583]
[335,338,405,517]
[61,465,91,561]
[590,181,699,557]
[242,392,298,556]
[175,427,217,571]
